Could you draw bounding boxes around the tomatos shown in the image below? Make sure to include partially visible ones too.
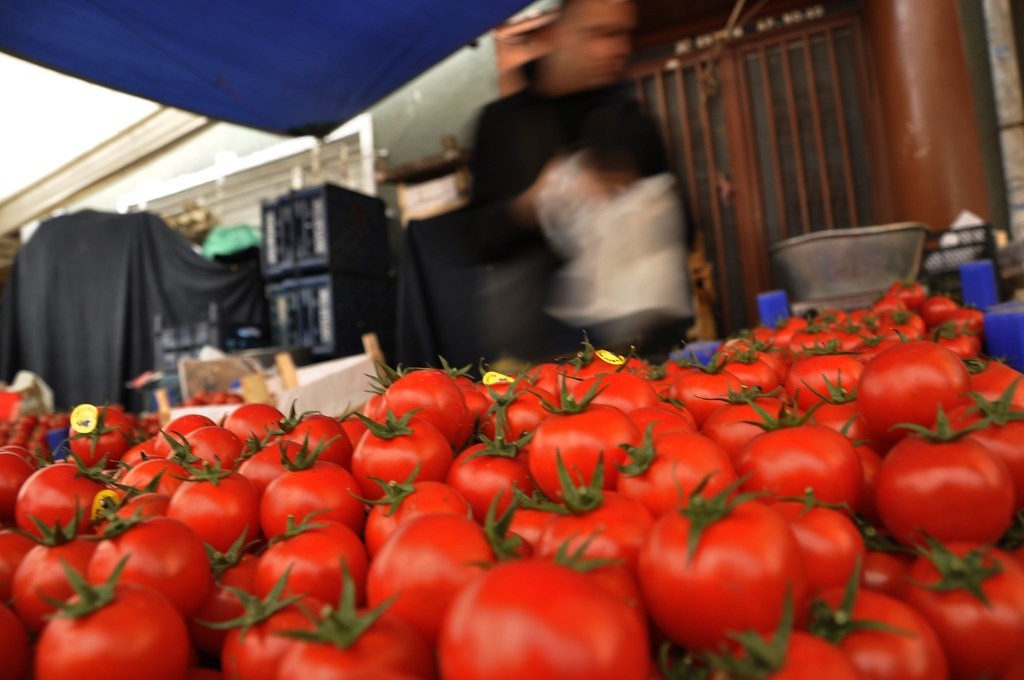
[0,282,1024,680]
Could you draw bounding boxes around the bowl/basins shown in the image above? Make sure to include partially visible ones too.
[767,222,929,302]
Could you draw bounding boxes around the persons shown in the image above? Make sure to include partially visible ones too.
[454,0,695,376]
